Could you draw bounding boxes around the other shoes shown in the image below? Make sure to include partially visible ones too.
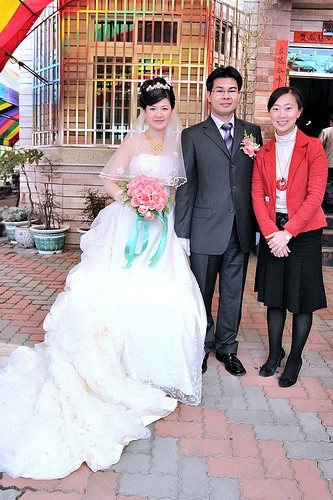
[322,206,333,213]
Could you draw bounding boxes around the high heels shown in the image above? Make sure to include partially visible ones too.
[259,348,285,377]
[279,358,302,387]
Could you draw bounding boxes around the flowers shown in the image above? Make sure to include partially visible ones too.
[120,175,173,221]
[239,129,261,159]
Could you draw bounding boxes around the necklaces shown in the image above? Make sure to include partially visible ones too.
[143,132,169,158]
[276,147,294,191]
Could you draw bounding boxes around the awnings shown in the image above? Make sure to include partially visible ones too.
[0,67,20,147]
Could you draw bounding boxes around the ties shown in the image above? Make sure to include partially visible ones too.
[221,123,233,156]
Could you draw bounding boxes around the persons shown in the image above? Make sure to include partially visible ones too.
[318,114,333,215]
[252,87,328,387]
[41,77,207,406]
[174,66,263,376]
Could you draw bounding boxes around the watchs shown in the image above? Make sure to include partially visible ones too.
[283,230,291,241]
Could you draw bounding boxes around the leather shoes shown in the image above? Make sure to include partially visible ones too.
[202,353,209,374]
[216,351,246,376]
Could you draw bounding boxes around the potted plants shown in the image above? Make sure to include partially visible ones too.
[0,143,70,255]
[76,187,114,243]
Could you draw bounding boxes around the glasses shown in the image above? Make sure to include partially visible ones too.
[211,87,238,97]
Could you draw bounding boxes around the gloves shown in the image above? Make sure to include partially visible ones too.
[178,238,190,256]
[256,232,260,245]
